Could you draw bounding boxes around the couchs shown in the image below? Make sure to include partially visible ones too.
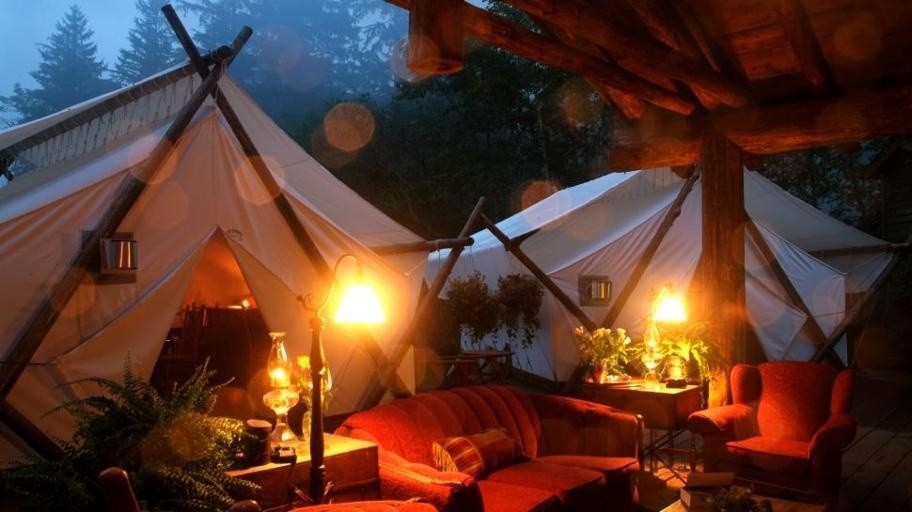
[330,384,645,512]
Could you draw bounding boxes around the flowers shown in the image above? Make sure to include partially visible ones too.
[576,328,631,370]
[448,269,543,349]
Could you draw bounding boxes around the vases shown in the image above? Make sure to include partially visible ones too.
[592,366,606,384]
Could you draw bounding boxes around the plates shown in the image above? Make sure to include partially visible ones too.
[583,375,632,385]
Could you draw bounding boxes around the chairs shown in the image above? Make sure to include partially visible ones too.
[160,305,207,377]
[688,360,859,512]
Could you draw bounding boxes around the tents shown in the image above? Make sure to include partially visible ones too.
[1,62,431,474]
[425,151,891,384]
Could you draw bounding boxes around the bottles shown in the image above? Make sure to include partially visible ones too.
[242,419,274,467]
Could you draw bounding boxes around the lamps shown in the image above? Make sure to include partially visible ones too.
[292,256,388,505]
[642,313,662,383]
[263,333,300,449]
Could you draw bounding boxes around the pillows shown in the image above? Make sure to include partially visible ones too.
[433,426,515,481]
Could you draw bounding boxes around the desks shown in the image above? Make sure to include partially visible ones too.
[584,377,708,489]
[226,431,380,512]
[416,352,514,390]
[204,308,269,387]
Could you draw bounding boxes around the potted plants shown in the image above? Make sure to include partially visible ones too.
[626,339,708,380]
